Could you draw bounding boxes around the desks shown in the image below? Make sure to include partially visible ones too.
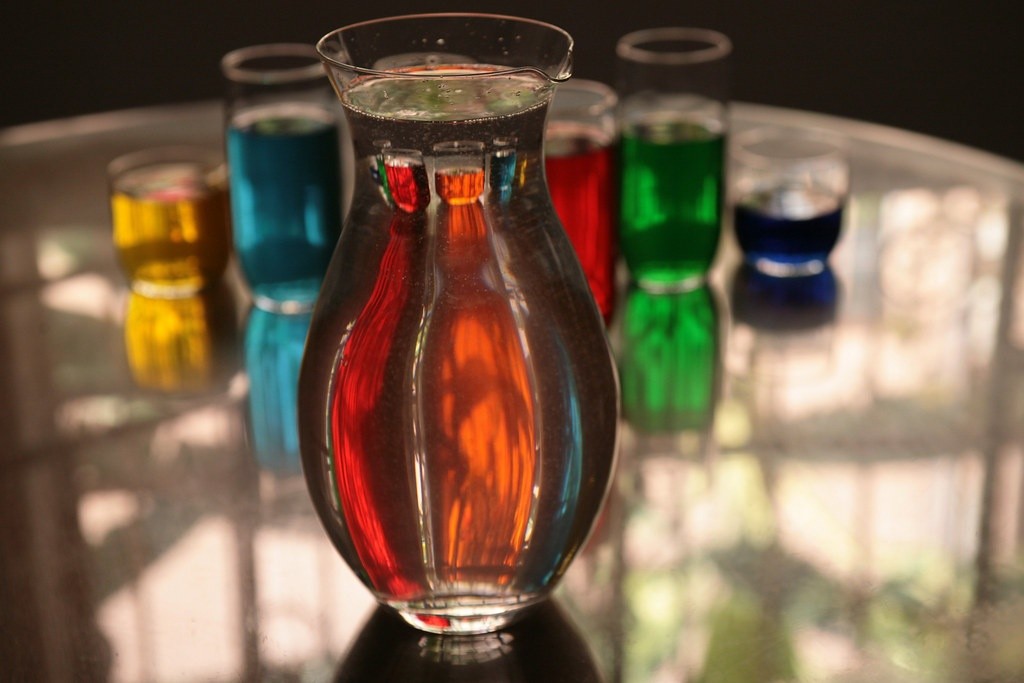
[0,88,1024,683]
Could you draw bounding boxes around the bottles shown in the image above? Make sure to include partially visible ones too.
[299,13,617,634]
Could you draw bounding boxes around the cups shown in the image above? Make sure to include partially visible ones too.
[109,144,231,299]
[224,42,348,311]
[541,81,614,322]
[728,124,852,278]
[239,307,338,519]
[125,296,226,417]
[616,28,735,294]
[726,269,845,407]
[613,281,726,508]
